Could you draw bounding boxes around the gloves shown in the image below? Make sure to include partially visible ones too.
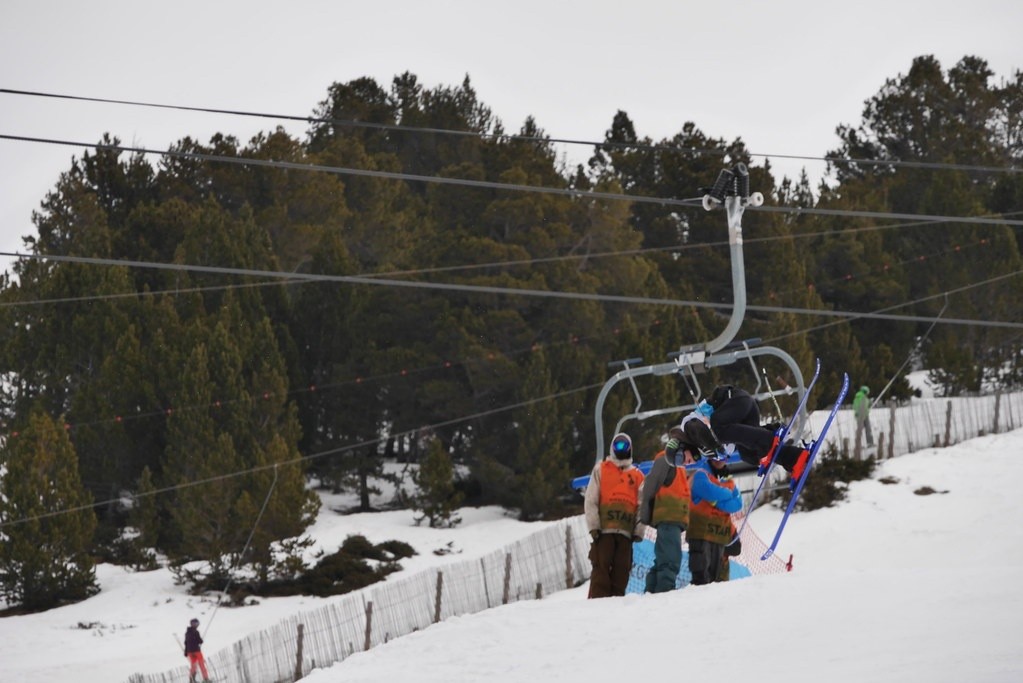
[590,529,600,540]
[634,536,642,542]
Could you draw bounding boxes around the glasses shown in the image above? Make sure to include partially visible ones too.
[674,446,685,467]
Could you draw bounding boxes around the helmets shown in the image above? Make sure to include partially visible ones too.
[665,439,690,468]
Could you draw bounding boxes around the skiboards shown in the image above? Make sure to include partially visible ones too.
[191,673,229,683]
[727,358,849,561]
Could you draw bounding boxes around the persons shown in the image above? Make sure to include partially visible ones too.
[665,384,811,490]
[184,618,212,683]
[640,425,691,594]
[854,386,876,450]
[685,460,743,585]
[583,433,647,598]
